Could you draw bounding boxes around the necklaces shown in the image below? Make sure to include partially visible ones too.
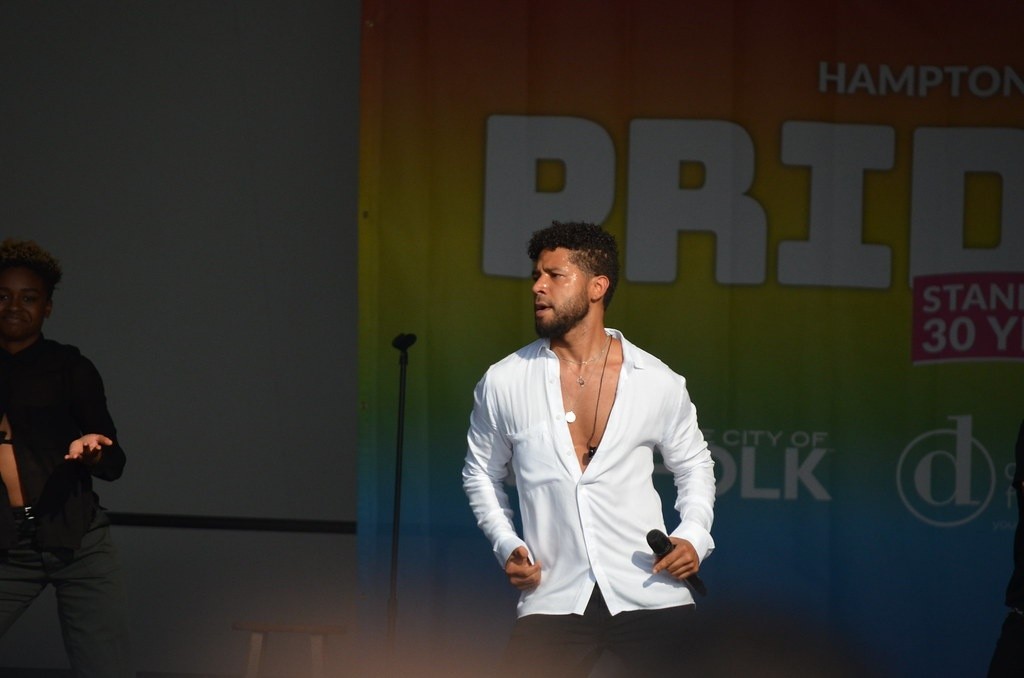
[549,336,609,423]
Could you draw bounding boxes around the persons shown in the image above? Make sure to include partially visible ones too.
[462,222,716,678]
[0,242,122,678]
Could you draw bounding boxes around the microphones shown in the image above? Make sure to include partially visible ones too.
[646,529,707,598]
[588,445,596,458]
